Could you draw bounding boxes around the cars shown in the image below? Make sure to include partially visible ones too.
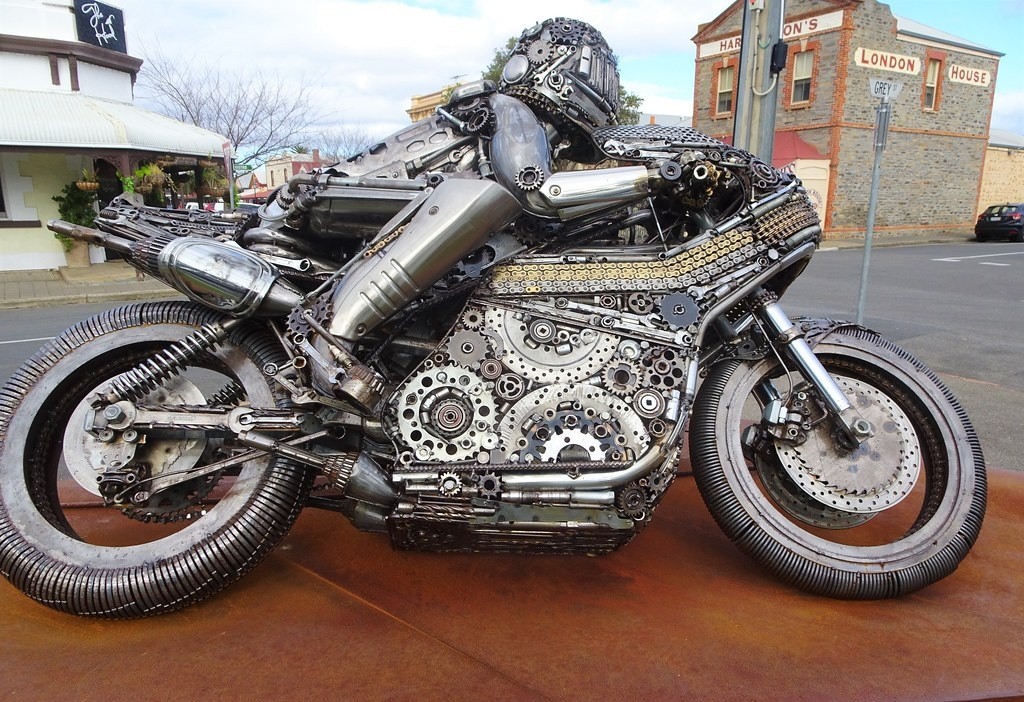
[975,201,1024,242]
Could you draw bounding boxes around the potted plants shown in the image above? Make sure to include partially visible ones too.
[131,162,170,197]
[196,168,241,210]
[75,166,102,194]
[51,181,101,270]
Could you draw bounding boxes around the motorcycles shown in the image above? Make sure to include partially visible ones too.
[0,124,991,624]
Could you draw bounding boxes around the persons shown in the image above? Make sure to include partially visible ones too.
[261,16,720,412]
